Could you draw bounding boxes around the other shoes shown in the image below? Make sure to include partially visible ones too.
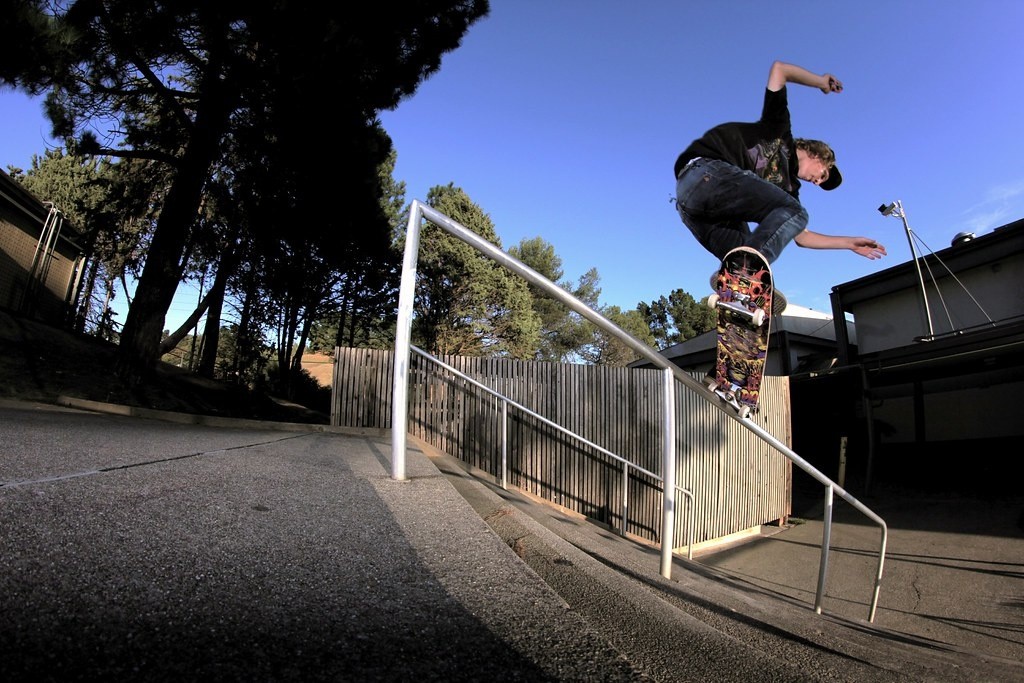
[710,268,786,316]
[702,366,760,414]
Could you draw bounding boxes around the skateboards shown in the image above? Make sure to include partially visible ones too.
[706,246,775,419]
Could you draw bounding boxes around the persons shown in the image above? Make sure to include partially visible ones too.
[674,61,887,413]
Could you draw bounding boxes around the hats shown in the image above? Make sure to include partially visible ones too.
[808,139,842,192]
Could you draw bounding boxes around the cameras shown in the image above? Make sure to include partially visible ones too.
[878,202,898,216]
